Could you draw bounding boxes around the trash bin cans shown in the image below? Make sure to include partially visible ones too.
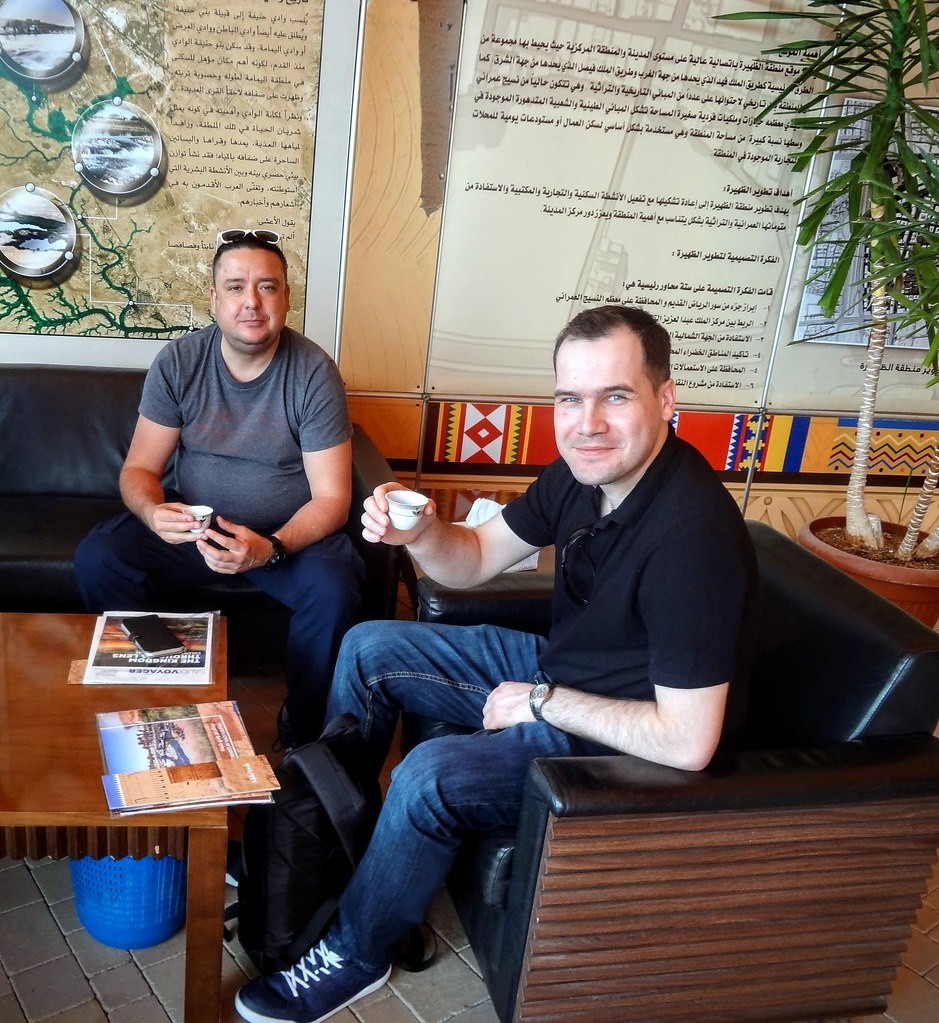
[69,855,188,950]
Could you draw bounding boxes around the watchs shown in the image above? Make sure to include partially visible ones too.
[529,683,553,721]
[266,533,285,571]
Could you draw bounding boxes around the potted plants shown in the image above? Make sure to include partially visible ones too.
[712,0,939,634]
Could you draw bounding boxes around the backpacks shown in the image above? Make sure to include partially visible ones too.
[220,716,438,972]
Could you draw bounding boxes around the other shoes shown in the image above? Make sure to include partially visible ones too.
[271,701,325,751]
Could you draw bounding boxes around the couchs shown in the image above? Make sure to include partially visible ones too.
[0,364,399,683]
[395,517,939,1022]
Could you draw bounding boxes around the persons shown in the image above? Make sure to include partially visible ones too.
[225,305,760,1023]
[76,238,368,759]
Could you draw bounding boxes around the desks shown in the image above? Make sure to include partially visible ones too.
[0,612,230,1022]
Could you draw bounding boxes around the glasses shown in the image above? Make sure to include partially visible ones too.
[215,228,283,256]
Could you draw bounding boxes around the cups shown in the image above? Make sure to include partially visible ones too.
[385,491,428,530]
[183,505,214,533]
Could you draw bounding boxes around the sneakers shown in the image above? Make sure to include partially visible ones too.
[234,937,392,1023]
